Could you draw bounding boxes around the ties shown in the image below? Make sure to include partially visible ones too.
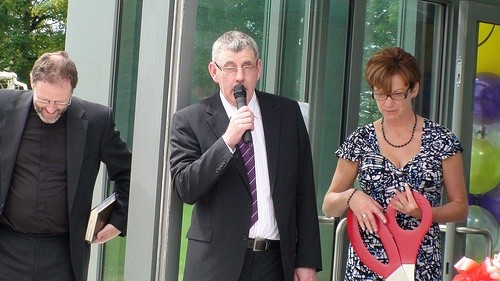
[238,136,258,229]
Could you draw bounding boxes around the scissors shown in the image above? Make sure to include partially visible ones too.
[347,188,433,281]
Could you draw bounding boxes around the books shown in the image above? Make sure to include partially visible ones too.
[84,191,119,243]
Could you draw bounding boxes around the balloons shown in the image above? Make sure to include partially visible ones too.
[464,204,500,266]
[466,138,500,196]
[466,69,500,129]
[468,194,500,222]
[476,20,500,77]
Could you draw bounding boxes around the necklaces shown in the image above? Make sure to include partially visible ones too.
[380,111,418,147]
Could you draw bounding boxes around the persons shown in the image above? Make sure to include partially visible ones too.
[166,28,323,281]
[322,47,468,281]
[0,52,133,281]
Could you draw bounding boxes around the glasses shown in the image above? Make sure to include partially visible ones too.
[372,87,410,101]
[215,60,258,75]
[33,87,72,107]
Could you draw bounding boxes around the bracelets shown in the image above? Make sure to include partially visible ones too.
[347,188,357,206]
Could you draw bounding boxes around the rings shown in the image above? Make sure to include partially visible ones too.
[399,205,404,210]
[362,215,367,218]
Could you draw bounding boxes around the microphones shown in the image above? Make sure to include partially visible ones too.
[233,84,252,144]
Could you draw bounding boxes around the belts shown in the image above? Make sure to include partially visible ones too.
[247,238,281,252]
